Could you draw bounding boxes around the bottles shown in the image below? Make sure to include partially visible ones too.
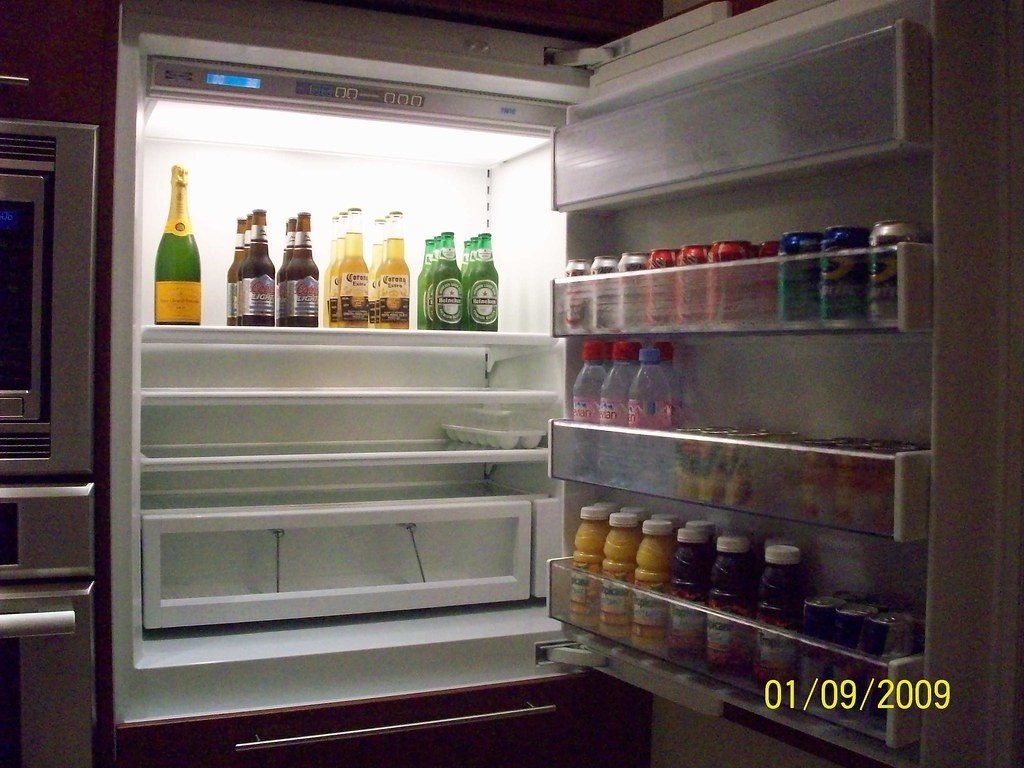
[323,207,410,330]
[226,208,319,327]
[572,340,697,489]
[568,503,802,689]
[154,165,201,326]
[417,231,499,332]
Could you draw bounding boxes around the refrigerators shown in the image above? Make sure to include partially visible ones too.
[103,1,1023,768]
